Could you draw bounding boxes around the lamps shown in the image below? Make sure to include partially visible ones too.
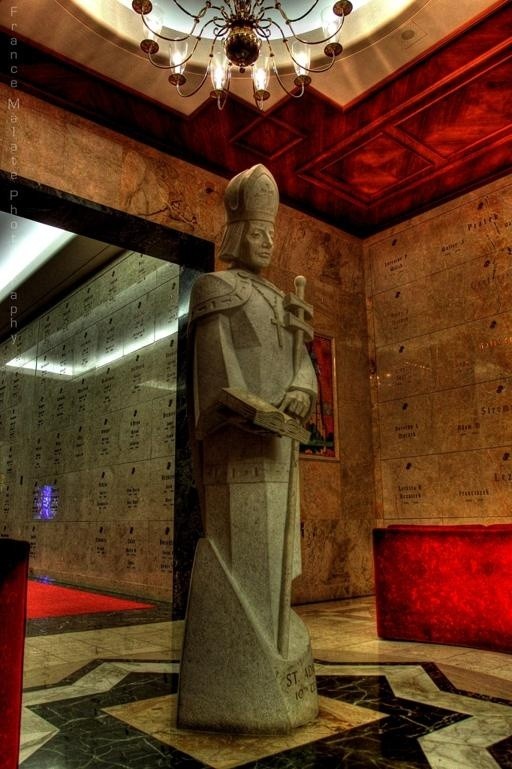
[130,0,355,110]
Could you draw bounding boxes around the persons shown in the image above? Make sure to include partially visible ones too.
[168,159,321,733]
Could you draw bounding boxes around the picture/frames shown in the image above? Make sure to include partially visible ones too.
[300,328,341,463]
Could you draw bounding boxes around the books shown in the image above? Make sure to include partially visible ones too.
[217,386,312,445]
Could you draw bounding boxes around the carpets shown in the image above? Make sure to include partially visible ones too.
[25,577,153,621]
